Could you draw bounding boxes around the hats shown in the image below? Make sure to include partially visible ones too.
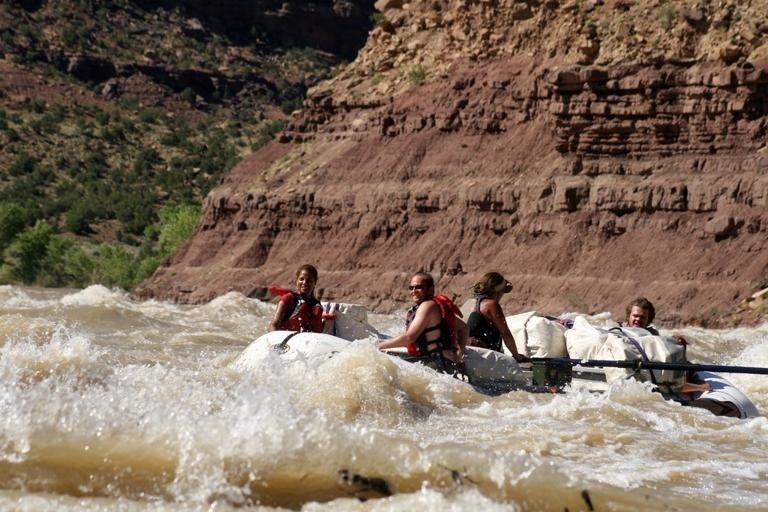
[489,278,513,293]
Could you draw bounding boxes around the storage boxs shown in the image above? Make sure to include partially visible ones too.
[528,361,572,387]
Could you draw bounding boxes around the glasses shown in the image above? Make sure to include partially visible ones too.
[408,284,431,290]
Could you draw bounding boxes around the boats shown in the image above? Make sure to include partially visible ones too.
[230,313,760,420]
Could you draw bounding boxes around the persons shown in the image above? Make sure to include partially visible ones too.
[556,296,688,349]
[265,264,329,334]
[460,269,525,365]
[378,271,468,376]
